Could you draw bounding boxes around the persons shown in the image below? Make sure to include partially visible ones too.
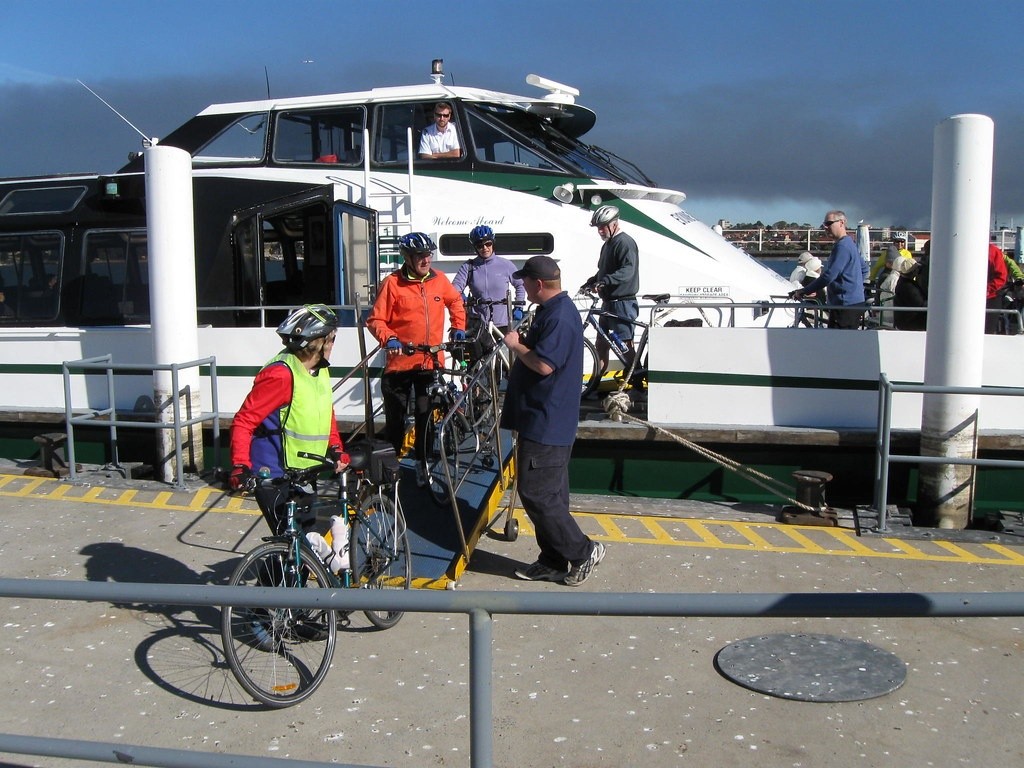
[870,225,1024,335]
[789,210,868,330]
[230,303,350,653]
[501,257,606,587]
[452,226,525,370]
[418,103,461,159]
[580,206,647,400]
[365,233,467,487]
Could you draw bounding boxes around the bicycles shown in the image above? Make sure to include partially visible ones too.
[220,440,414,710]
[785,292,897,331]
[402,298,525,510]
[580,284,670,399]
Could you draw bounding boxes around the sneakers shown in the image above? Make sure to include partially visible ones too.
[515,560,568,581]
[564,541,606,585]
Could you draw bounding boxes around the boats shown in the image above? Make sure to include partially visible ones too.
[0,60,1024,512]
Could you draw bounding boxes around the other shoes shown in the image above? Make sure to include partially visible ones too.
[586,391,598,399]
[242,620,280,651]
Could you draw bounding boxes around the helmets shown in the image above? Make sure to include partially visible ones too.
[589,205,619,226]
[276,304,337,338]
[469,225,495,244]
[399,232,437,253]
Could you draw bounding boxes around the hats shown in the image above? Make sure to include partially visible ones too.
[798,253,813,263]
[512,256,560,280]
[805,260,821,271]
[893,257,904,271]
[901,258,921,273]
[893,231,906,240]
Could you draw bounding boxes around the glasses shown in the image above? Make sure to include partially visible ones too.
[477,241,493,249]
[332,335,335,343]
[435,112,449,117]
[415,252,434,258]
[895,239,904,243]
[823,221,838,226]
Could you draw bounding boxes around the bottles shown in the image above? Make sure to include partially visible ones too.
[498,339,516,366]
[307,532,339,573]
[609,329,629,355]
[331,516,350,568]
[448,381,466,410]
